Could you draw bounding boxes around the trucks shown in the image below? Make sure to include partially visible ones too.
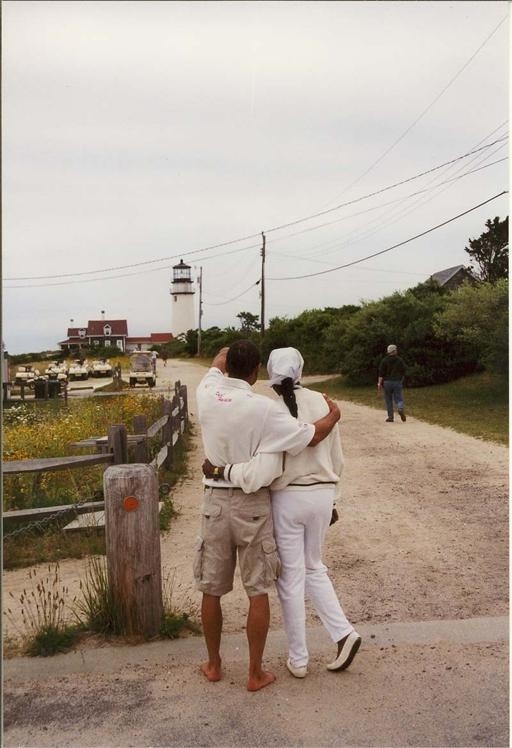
[129,349,157,388]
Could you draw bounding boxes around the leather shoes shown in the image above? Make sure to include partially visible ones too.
[285,657,308,678]
[325,629,361,673]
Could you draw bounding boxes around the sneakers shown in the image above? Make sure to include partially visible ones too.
[385,409,407,422]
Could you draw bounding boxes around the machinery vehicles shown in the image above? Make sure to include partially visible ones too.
[15,357,114,390]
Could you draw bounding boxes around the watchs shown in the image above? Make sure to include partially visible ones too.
[213,467,220,478]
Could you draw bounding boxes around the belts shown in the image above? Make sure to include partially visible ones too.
[204,483,270,492]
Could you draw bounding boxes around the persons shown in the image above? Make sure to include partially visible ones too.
[201,346,362,678]
[377,344,408,422]
[191,340,341,692]
[161,346,168,368]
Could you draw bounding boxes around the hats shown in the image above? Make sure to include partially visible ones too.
[386,343,397,353]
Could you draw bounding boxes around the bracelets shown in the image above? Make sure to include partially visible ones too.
[377,383,382,386]
